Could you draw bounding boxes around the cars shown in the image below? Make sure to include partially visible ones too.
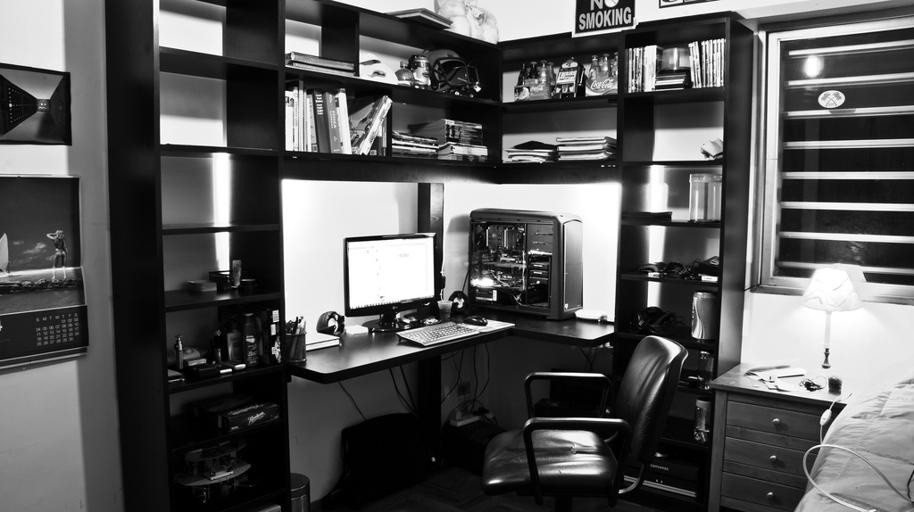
[437,301,454,321]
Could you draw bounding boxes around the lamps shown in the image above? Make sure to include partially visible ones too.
[483,333,689,512]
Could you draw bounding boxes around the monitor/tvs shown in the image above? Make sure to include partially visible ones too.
[305,332,340,353]
[284,80,488,163]
[285,60,356,78]
[384,7,454,31]
[627,38,724,93]
[285,51,355,72]
[504,134,618,165]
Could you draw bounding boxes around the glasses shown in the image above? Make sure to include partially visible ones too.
[800,267,863,392]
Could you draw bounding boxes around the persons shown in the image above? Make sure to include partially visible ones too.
[46,230,69,282]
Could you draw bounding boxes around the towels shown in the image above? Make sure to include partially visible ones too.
[290,474,310,512]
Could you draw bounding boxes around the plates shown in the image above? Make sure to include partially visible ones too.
[496,30,621,170]
[62,1,293,511]
[226,2,498,168]
[613,12,753,511]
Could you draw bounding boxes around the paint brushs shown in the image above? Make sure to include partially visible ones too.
[449,410,494,427]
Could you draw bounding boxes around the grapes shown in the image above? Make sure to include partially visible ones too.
[707,363,848,511]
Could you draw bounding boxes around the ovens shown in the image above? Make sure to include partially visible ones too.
[795,371,914,512]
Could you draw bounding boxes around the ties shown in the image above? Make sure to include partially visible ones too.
[342,233,439,331]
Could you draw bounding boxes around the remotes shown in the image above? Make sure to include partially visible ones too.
[463,315,487,326]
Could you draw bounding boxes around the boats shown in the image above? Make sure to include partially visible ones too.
[448,291,468,316]
[317,311,345,337]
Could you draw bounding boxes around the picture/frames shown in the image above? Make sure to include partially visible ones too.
[293,320,615,426]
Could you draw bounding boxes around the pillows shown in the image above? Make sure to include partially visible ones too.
[424,51,479,97]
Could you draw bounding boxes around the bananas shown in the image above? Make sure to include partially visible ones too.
[395,321,480,347]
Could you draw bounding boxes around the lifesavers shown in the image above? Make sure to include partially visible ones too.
[467,207,584,321]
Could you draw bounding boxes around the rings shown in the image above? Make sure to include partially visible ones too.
[286,317,306,363]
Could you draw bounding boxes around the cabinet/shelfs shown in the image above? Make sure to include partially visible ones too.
[242,311,259,366]
[519,60,555,83]
[589,52,618,79]
